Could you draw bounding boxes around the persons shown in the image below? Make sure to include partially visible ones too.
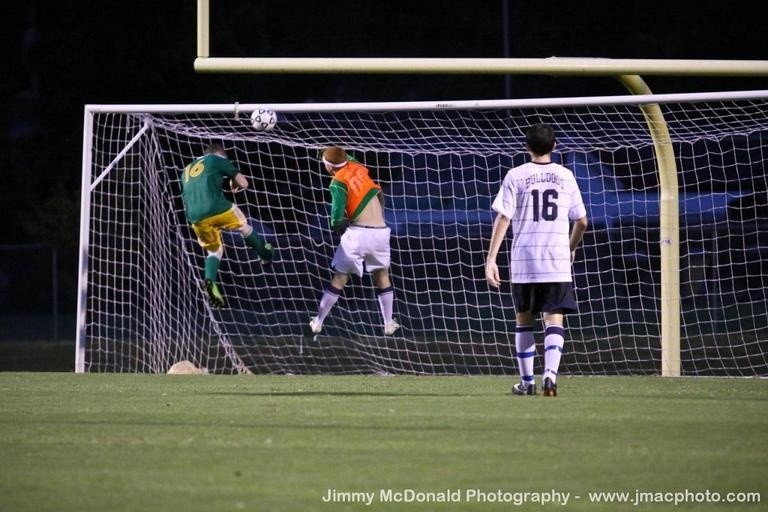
[179,143,277,307]
[485,125,589,397]
[306,145,400,335]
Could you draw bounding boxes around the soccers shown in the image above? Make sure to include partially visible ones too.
[250,110,278,131]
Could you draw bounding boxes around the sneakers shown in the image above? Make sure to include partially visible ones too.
[513,381,536,395]
[309,317,322,333]
[203,279,225,306]
[541,377,556,396]
[262,243,274,265]
[384,322,400,335]
